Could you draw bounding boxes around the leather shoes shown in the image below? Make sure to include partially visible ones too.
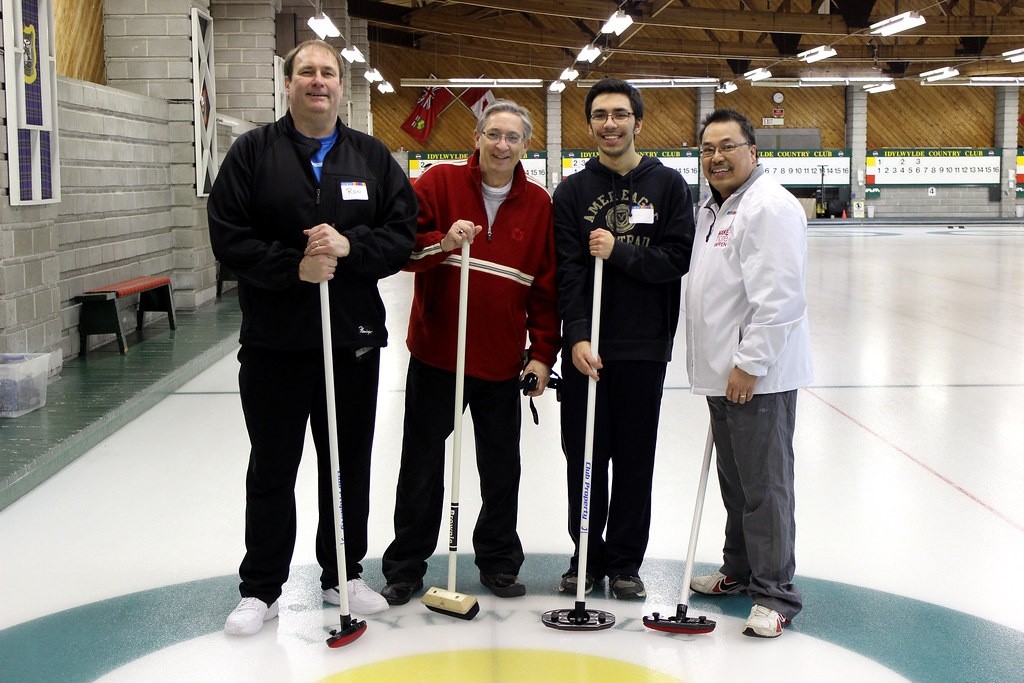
[380,578,423,605]
[480,572,526,597]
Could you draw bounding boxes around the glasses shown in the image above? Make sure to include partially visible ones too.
[589,112,636,124]
[702,142,750,155]
[479,130,528,145]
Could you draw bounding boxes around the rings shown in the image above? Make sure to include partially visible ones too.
[316,241,319,247]
[740,394,746,398]
[458,230,463,235]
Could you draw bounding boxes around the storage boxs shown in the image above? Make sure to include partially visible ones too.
[0,353,49,418]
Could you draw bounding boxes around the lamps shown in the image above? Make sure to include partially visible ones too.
[307,10,397,94]
[863,9,1024,94]
[400,77,544,87]
[797,43,837,63]
[549,8,772,93]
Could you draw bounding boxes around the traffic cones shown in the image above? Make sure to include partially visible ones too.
[841,210,846,220]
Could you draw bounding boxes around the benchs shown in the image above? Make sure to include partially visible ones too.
[74,275,178,356]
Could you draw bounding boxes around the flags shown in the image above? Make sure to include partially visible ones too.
[461,76,497,121]
[400,76,452,150]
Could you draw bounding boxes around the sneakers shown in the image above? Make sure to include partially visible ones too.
[742,605,791,638]
[224,597,279,635]
[610,573,646,599]
[322,578,389,615]
[690,572,747,595]
[559,560,593,594]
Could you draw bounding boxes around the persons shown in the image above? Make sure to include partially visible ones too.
[812,190,827,217]
[685,108,816,638]
[550,77,695,599]
[206,38,418,635]
[380,98,561,606]
